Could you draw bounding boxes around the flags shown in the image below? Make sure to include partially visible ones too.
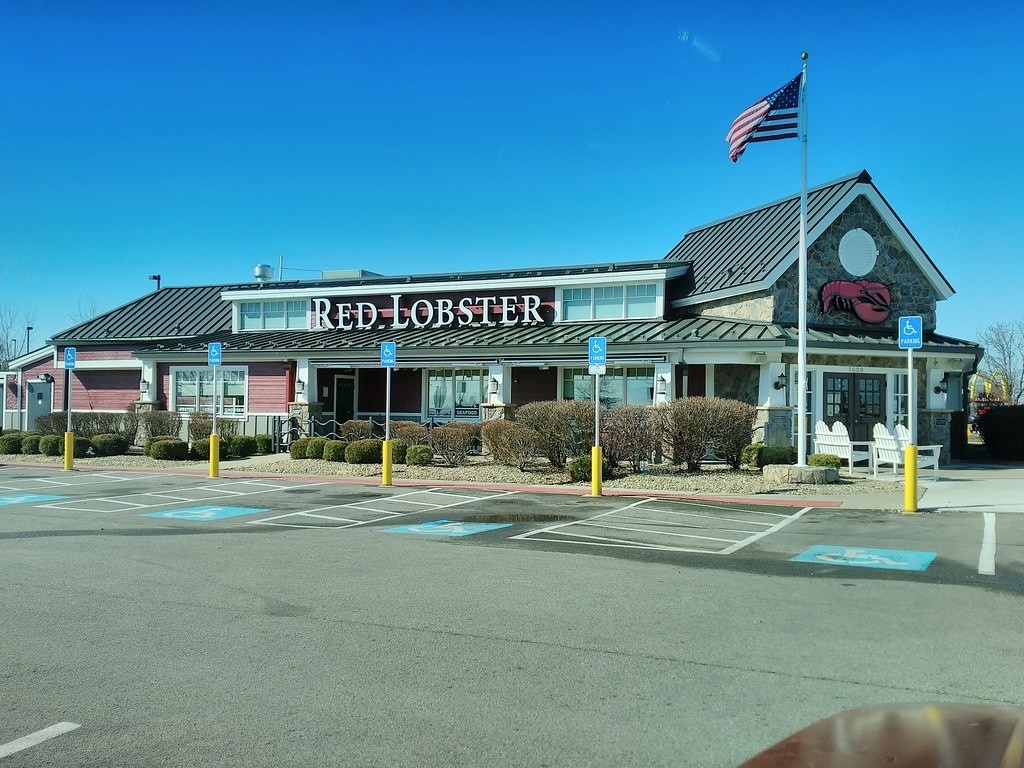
[726,72,803,163]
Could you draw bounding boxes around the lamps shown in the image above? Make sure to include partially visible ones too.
[489,377,498,394]
[656,375,666,394]
[295,379,305,393]
[752,352,766,363]
[773,372,786,390]
[951,359,965,372]
[140,379,150,394]
[934,377,949,394]
[38,373,55,384]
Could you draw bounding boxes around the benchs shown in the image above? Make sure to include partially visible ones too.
[813,419,875,475]
[871,423,943,481]
